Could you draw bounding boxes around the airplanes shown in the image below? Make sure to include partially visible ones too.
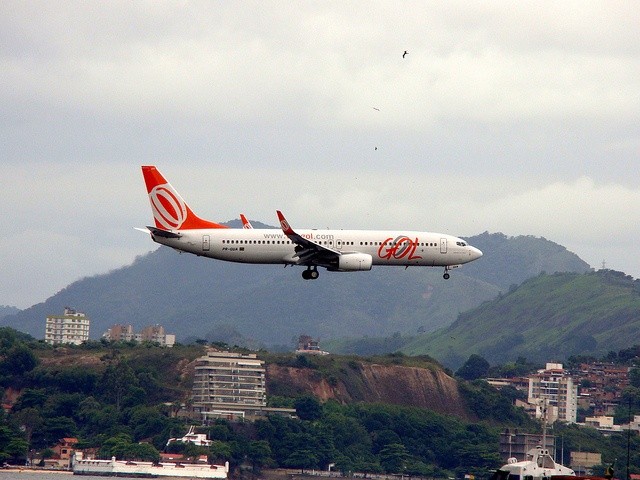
[134,165,483,280]
